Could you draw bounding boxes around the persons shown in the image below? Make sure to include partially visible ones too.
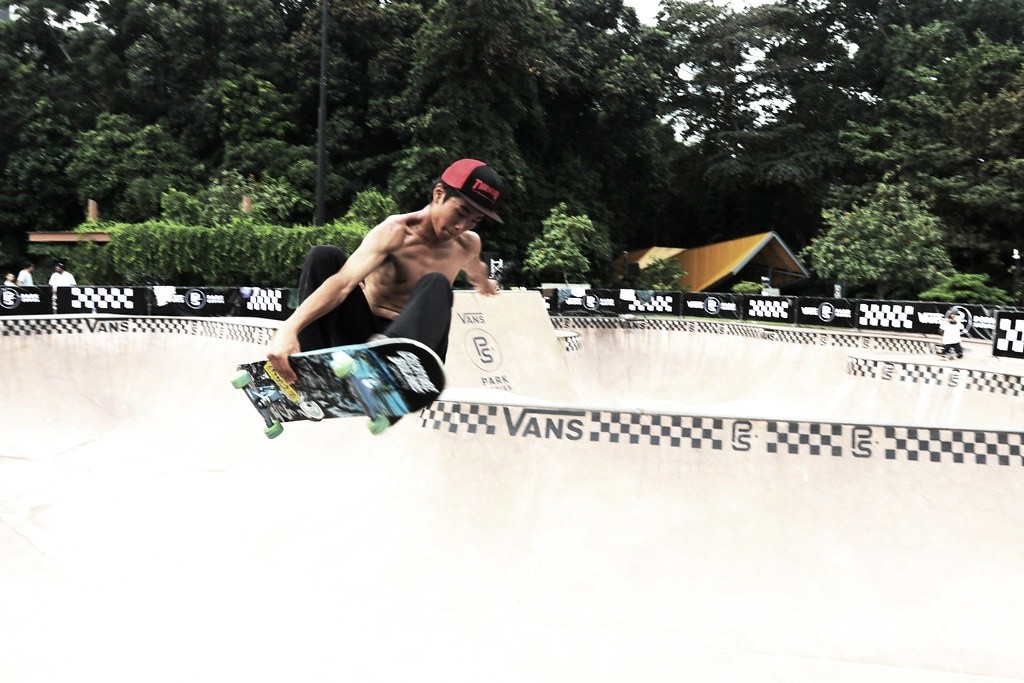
[267,158,505,429]
[48,263,78,286]
[4,273,15,286]
[936,307,964,359]
[16,262,34,286]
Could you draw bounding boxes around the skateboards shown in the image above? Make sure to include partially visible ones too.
[226,335,449,442]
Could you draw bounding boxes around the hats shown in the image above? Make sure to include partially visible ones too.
[443,158,504,224]
